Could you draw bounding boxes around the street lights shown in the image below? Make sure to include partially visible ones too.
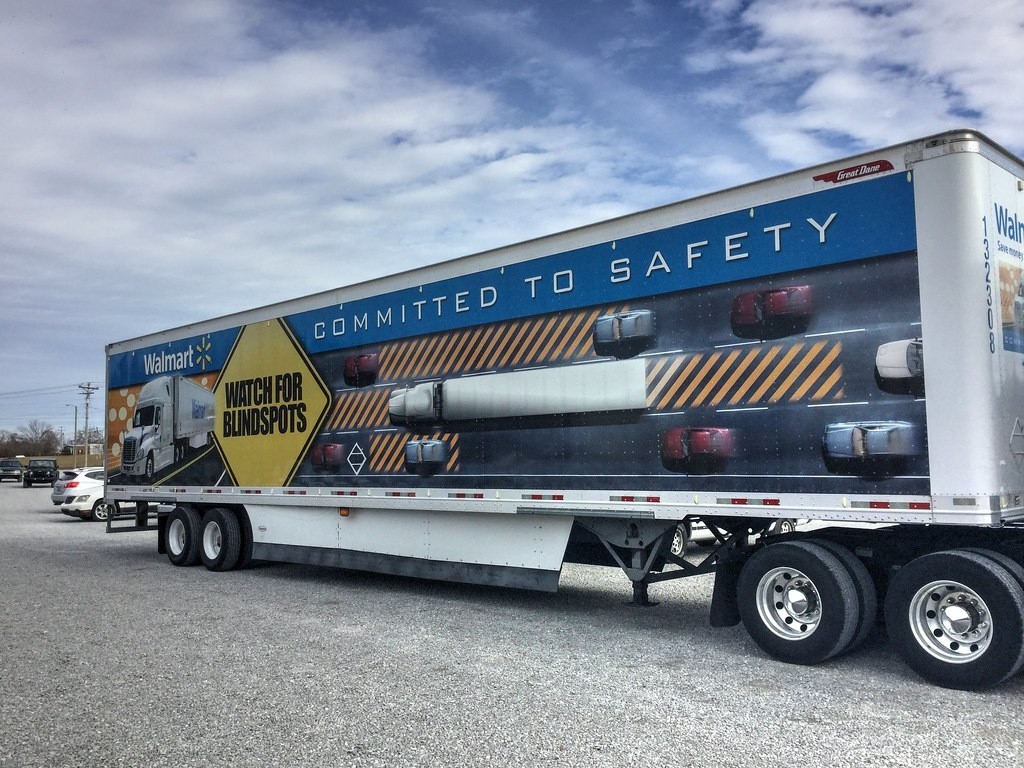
[66,404,78,469]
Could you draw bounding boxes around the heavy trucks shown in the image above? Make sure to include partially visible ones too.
[106,129,1024,692]
[119,375,217,481]
[388,357,647,425]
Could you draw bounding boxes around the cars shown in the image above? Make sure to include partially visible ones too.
[51,467,105,504]
[60,484,162,522]
[733,284,815,332]
[405,441,449,466]
[343,354,378,379]
[593,309,659,347]
[823,422,919,461]
[670,517,813,557]
[875,336,923,381]
[311,445,346,465]
[663,428,734,459]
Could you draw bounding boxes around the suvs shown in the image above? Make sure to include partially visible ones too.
[0,459,23,482]
[23,459,60,488]
